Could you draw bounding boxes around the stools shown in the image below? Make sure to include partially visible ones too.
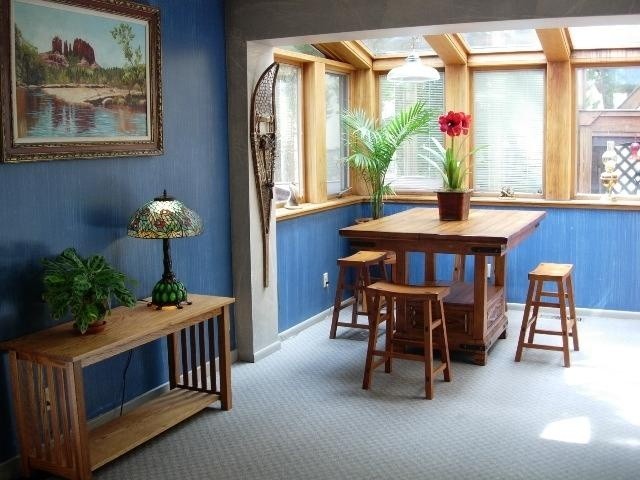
[330,251,395,343]
[362,280,452,399]
[516,262,579,367]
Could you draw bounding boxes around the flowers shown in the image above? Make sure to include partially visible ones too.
[418,110,491,190]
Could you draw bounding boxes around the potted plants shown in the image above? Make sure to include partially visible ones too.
[39,245,139,336]
[338,97,440,259]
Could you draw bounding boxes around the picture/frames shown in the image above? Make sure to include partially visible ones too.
[0,0,165,164]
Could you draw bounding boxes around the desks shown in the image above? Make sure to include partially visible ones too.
[339,206,547,366]
[0,293,235,480]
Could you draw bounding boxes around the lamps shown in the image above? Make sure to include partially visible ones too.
[386,35,441,83]
[128,187,203,311]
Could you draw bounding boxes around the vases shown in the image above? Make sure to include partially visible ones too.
[435,189,474,220]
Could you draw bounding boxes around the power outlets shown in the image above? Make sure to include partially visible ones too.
[323,272,329,288]
